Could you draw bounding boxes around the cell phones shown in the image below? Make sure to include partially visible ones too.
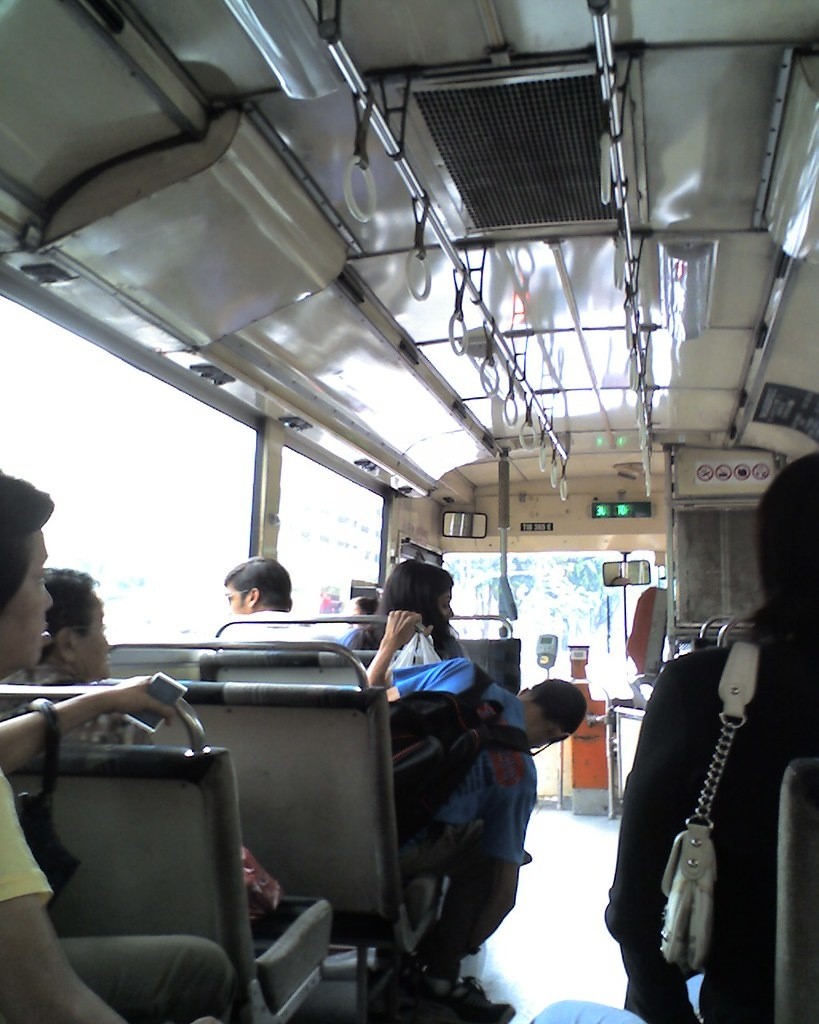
[122,673,187,734]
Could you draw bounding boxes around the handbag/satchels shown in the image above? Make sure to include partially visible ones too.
[662,640,760,977]
[241,845,285,924]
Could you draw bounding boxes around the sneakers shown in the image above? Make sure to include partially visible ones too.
[373,958,516,1024]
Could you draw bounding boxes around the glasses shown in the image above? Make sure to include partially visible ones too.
[225,589,251,606]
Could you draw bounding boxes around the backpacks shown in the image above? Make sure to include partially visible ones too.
[389,662,532,850]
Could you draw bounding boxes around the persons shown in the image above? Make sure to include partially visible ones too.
[0,454,819,1024]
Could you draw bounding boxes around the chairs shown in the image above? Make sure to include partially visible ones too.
[0,611,520,1024]
